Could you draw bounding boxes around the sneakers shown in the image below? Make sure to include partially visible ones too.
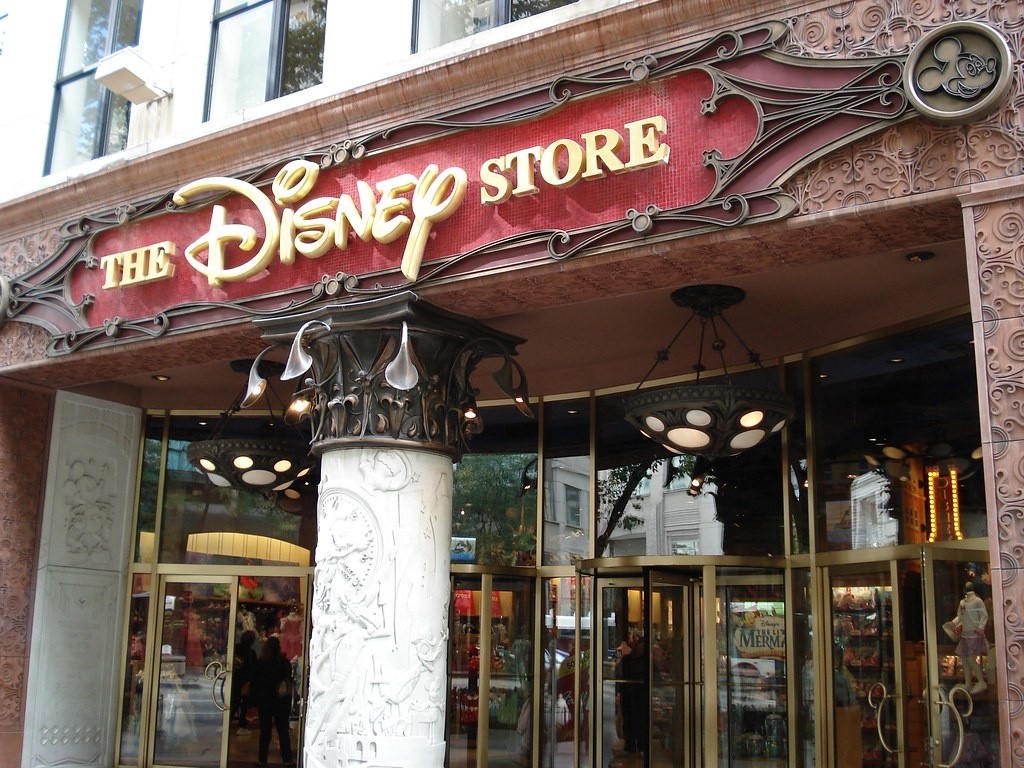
[971,682,988,694]
[959,683,973,694]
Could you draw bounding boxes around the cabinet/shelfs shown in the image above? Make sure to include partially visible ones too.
[809,570,905,768]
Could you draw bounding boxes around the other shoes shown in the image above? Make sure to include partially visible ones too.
[255,763,267,768]
[236,727,253,736]
[284,761,297,768]
[216,725,223,732]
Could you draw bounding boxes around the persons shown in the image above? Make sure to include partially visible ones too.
[549,639,588,734]
[232,633,297,768]
[614,637,658,752]
[279,612,303,661]
[946,591,989,694]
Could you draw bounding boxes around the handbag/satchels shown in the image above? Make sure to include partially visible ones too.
[615,700,625,738]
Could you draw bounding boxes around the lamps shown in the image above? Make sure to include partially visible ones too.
[496,353,534,419]
[620,284,797,460]
[480,337,516,404]
[519,457,537,497]
[848,437,984,488]
[385,320,418,389]
[283,371,315,426]
[239,343,287,409]
[185,360,318,496]
[279,320,331,380]
[688,456,707,496]
[464,406,476,419]
[662,458,679,487]
[283,475,308,500]
